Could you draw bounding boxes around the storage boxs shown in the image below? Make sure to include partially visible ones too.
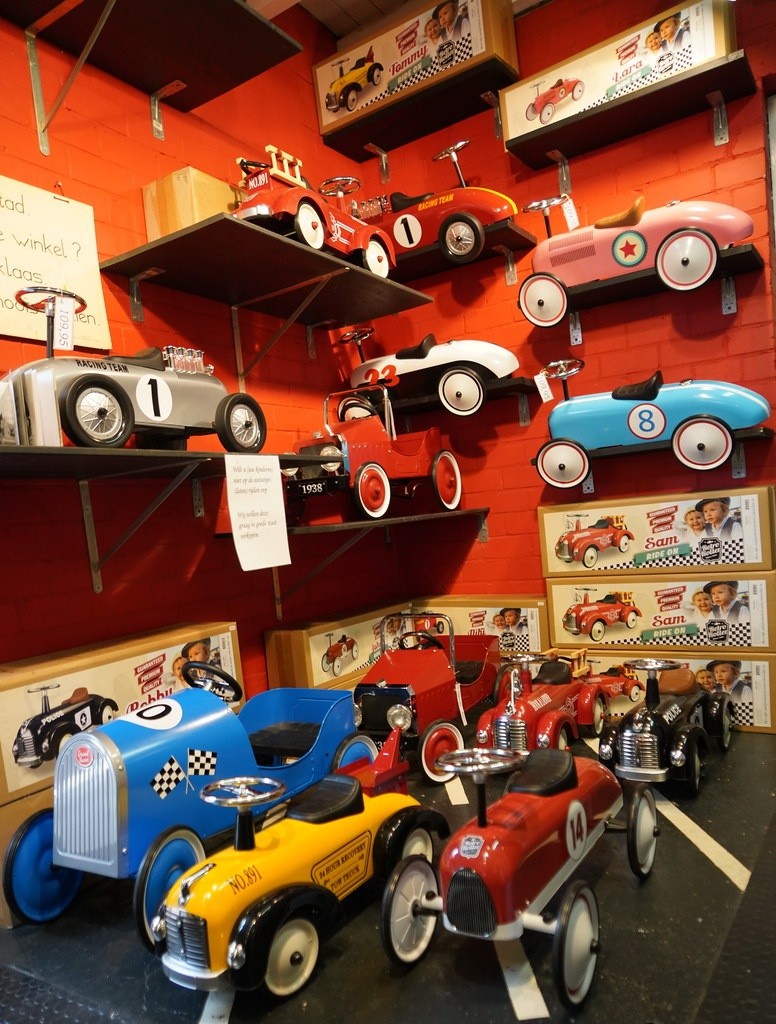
[536,483,776,573]
[503,3,739,155]
[550,648,776,735]
[310,0,521,140]
[409,594,551,659]
[546,570,776,655]
[0,783,56,929]
[0,620,248,804]
[141,166,250,244]
[265,597,418,690]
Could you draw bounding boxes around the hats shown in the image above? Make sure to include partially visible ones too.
[702,581,739,594]
[171,651,182,670]
[643,28,654,46]
[181,637,211,658]
[432,0,459,19]
[652,11,681,32]
[683,505,696,520]
[694,497,731,512]
[705,660,741,673]
[491,612,500,624]
[690,587,704,601]
[695,664,707,676]
[422,16,432,36]
[500,607,521,616]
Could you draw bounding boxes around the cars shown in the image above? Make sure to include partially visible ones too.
[0,661,379,956]
[523,73,587,125]
[318,46,387,114]
[227,145,397,279]
[281,384,462,520]
[148,773,453,1000]
[554,513,635,568]
[536,361,771,488]
[377,746,661,1007]
[12,682,120,769]
[360,140,517,266]
[351,610,739,797]
[0,284,267,454]
[339,328,520,418]
[561,588,643,642]
[321,633,360,676]
[517,193,755,328]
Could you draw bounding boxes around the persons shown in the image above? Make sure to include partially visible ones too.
[691,579,750,627]
[171,637,222,692]
[492,608,529,637]
[644,11,690,65]
[684,497,743,547]
[424,0,470,56]
[386,617,406,646]
[694,659,752,703]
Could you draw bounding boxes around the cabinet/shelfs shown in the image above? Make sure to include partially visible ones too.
[0,212,776,622]
